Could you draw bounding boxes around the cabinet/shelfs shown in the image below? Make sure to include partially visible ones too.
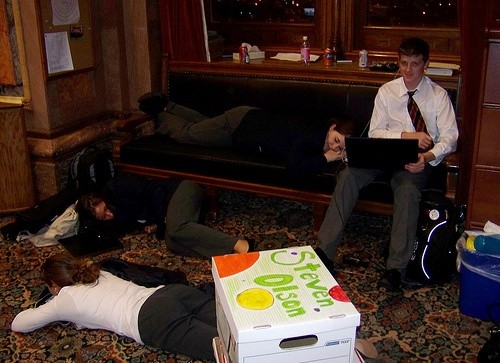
[457,39,500,231]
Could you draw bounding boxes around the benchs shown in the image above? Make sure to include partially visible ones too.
[112,61,462,239]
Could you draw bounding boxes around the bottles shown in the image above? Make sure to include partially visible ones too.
[474,235,500,255]
[301,36,310,66]
[240,43,248,63]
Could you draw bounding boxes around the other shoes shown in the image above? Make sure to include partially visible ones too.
[137,92,170,120]
[247,238,256,252]
[380,268,402,292]
[315,246,334,274]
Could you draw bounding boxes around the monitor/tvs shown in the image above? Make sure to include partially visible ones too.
[345,136,419,172]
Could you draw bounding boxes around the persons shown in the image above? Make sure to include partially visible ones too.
[12,253,217,361]
[138,92,356,171]
[67,175,254,258]
[314,37,459,290]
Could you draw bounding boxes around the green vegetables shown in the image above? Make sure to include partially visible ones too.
[369,63,400,72]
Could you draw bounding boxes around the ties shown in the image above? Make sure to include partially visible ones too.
[407,89,434,153]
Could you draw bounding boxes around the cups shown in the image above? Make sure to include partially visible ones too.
[323,50,336,65]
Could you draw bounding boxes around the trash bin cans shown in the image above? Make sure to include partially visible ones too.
[456,231,500,320]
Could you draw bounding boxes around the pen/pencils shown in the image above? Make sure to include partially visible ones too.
[337,59,353,63]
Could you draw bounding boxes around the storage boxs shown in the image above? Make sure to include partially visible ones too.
[455,232,500,326]
[211,245,361,363]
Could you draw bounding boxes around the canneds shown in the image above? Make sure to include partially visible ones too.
[323,48,333,66]
[239,46,248,62]
[358,49,368,68]
